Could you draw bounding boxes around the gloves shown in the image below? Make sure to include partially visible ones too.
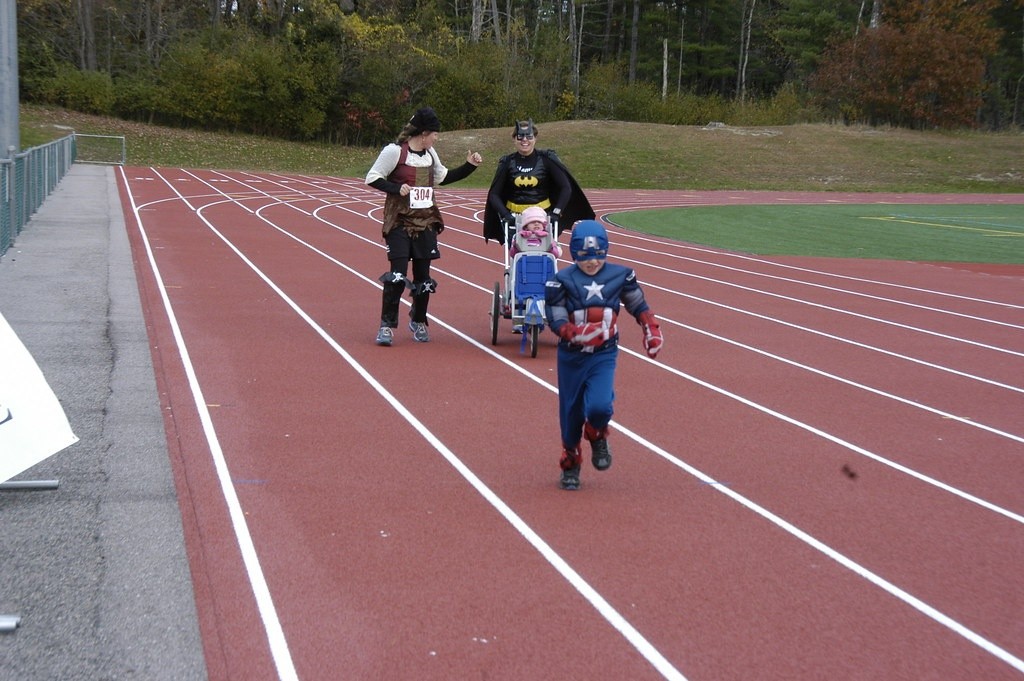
[535,230,549,242]
[503,215,515,226]
[549,211,560,223]
[559,321,604,347]
[639,310,663,359]
[518,231,533,238]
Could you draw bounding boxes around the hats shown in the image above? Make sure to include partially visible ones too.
[521,206,547,229]
[410,107,441,132]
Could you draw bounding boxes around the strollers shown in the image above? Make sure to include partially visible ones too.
[488,212,560,360]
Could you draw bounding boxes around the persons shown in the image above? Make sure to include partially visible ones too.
[544,220,664,490]
[365,105,482,345]
[483,117,596,333]
[509,206,562,260]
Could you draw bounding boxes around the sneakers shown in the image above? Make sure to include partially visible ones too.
[561,463,580,490]
[376,325,393,346]
[409,319,428,342]
[590,438,612,470]
